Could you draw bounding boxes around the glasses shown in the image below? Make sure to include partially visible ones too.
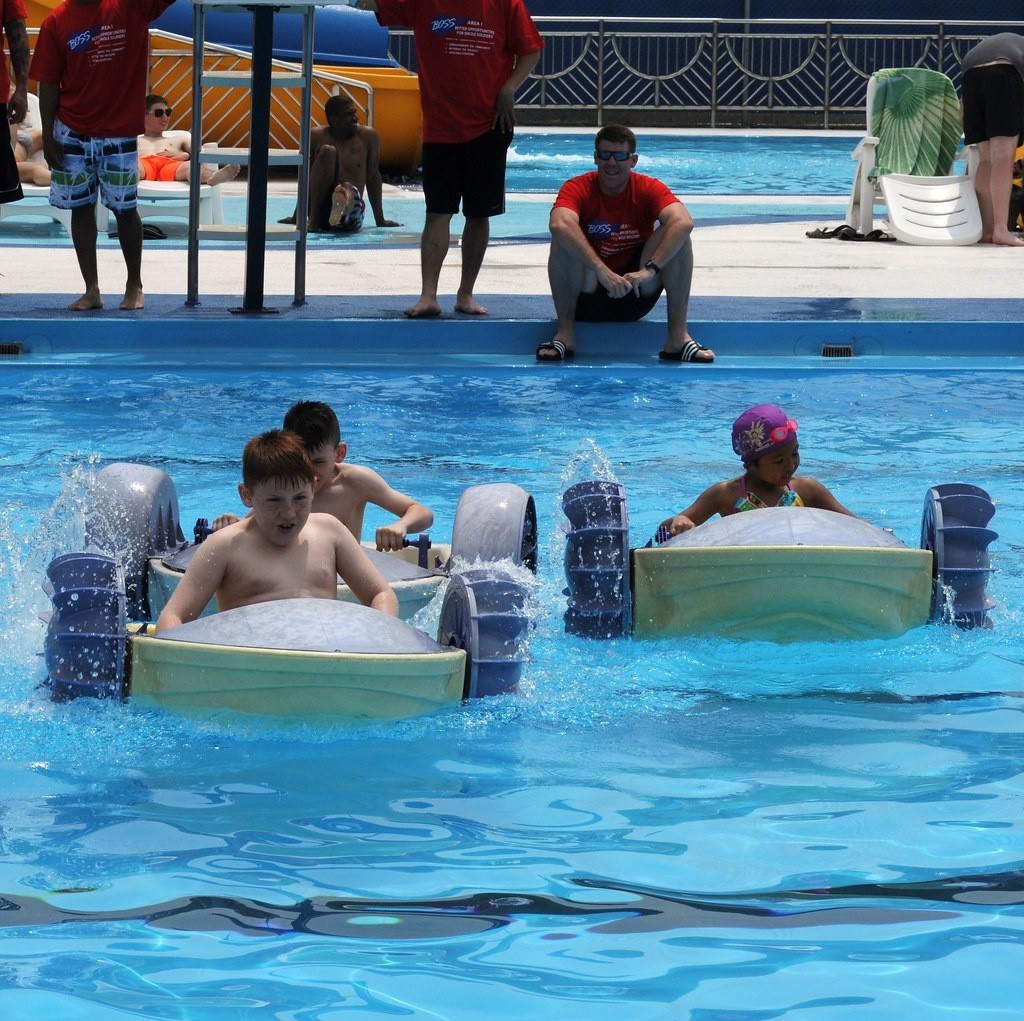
[769,419,799,444]
[597,149,635,162]
[146,108,173,117]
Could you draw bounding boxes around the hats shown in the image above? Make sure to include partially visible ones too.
[731,404,797,461]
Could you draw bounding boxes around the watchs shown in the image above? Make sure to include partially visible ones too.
[645,260,660,274]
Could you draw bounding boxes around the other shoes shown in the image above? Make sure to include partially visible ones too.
[109,224,167,239]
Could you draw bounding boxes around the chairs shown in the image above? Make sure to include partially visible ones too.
[0,93,226,240]
[845,68,984,247]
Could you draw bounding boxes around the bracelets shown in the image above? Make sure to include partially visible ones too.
[184,150,191,161]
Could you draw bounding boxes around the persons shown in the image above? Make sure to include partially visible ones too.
[655,404,872,536]
[536,125,716,364]
[212,400,434,552]
[352,0,545,317]
[958,33,1024,246]
[28,0,177,311]
[9,122,52,186]
[137,95,241,186]
[277,95,404,234]
[0,0,30,204]
[156,428,399,632]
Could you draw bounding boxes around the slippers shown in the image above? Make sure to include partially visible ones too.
[805,225,848,238]
[845,225,897,241]
[661,339,715,364]
[537,340,575,363]
